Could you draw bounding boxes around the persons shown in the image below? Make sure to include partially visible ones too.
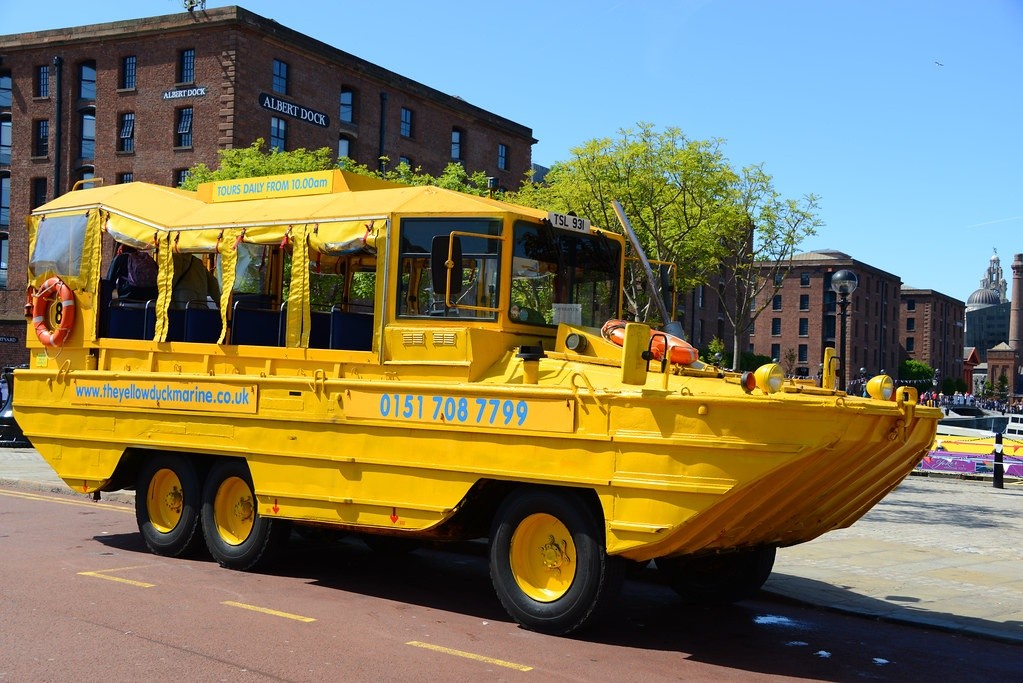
[920,390,969,406]
[108,245,221,310]
[846,385,870,398]
[974,398,1023,416]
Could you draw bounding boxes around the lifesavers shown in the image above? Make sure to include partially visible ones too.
[32,277,76,348]
[602,318,698,366]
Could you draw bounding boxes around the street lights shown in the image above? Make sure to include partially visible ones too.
[830,269,858,392]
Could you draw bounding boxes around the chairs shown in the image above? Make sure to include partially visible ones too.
[99,277,373,352]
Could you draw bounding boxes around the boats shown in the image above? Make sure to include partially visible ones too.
[937,412,1023,434]
